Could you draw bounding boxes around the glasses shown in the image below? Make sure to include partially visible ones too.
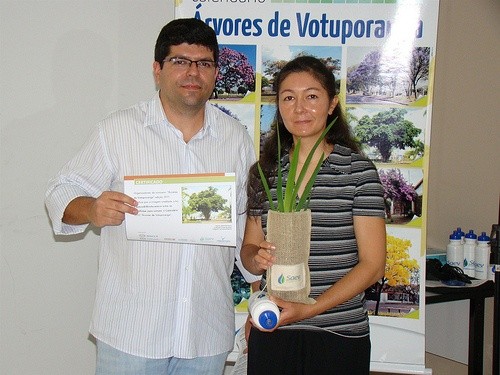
[163,56,217,71]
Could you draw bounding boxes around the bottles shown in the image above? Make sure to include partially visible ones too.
[456,228,464,245]
[247,291,280,330]
[446,230,464,275]
[464,230,477,277]
[474,232,490,280]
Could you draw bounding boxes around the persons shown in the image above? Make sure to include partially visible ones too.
[237,57,388,375]
[46,19,257,375]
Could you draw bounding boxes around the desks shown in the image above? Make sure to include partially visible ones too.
[425,270,500,375]
